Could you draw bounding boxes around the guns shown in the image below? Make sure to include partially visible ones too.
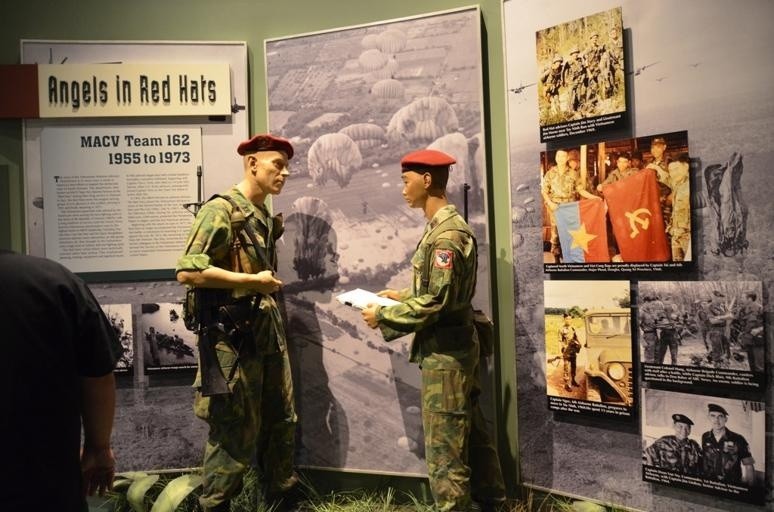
[547,355,577,363]
[723,294,737,339]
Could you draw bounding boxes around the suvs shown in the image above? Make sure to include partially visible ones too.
[582,306,634,407]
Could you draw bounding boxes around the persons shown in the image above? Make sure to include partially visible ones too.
[173,133,300,512]
[541,137,691,263]
[642,413,702,478]
[638,289,764,374]
[540,25,624,115]
[0,247,123,511]
[360,149,482,511]
[701,403,756,488]
[560,311,582,392]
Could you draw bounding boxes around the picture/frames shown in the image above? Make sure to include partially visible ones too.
[262,3,500,480]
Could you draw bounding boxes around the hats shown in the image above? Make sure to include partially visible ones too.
[671,414,693,425]
[238,134,294,160]
[607,27,617,36]
[713,290,725,298]
[567,150,580,161]
[652,138,665,145]
[616,151,630,160]
[708,403,728,415]
[569,47,580,55]
[402,150,456,172]
[590,32,599,40]
[552,56,562,64]
